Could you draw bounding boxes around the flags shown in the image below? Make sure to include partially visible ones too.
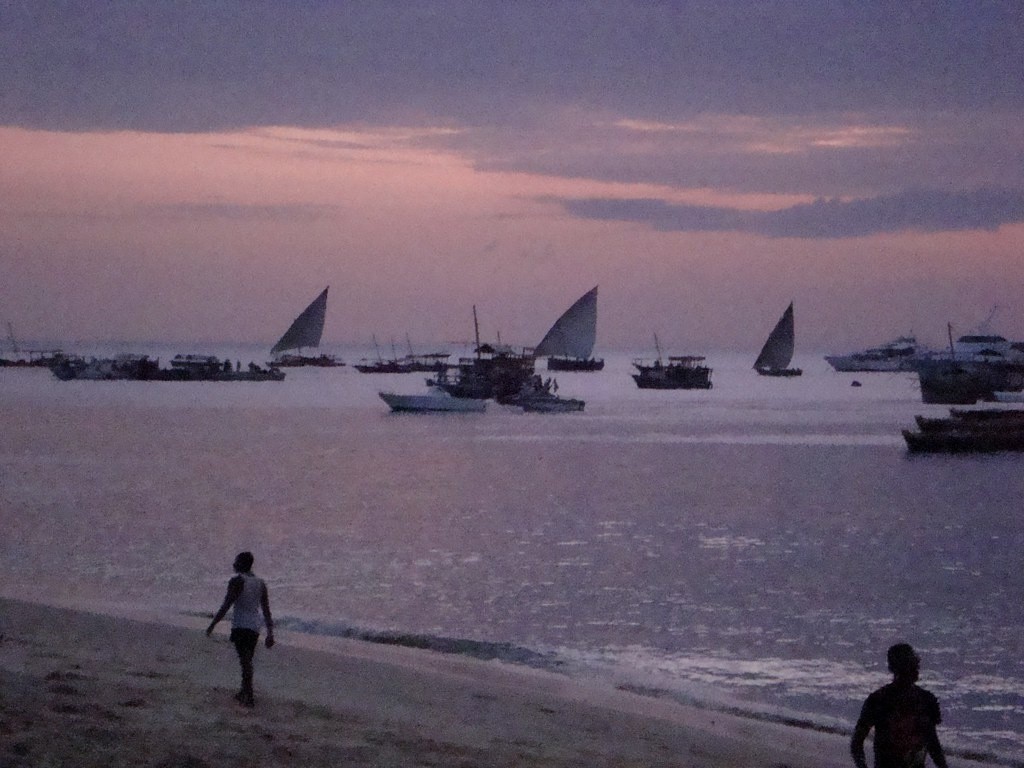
[554,379,558,392]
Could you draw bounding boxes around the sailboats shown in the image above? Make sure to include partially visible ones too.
[751,301,803,376]
[264,286,344,365]
[533,285,607,370]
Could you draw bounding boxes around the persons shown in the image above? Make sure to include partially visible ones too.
[206,551,274,709]
[851,643,949,768]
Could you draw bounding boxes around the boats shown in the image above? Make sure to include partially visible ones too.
[825,336,928,372]
[378,385,486,412]
[631,332,715,389]
[354,305,585,411]
[902,400,1024,453]
[47,349,284,382]
[916,322,1024,402]
